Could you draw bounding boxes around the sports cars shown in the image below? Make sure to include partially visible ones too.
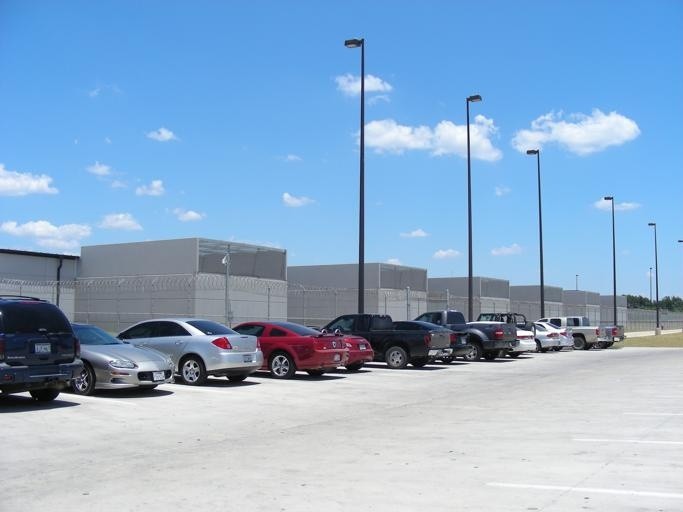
[307,326,374,371]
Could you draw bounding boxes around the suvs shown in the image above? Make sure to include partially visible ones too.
[0,294,84,402]
[467,313,537,358]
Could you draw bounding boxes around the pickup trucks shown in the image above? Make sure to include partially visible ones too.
[412,310,517,362]
[536,316,626,351]
[320,313,474,370]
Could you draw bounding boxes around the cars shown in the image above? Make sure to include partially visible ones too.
[230,321,349,380]
[517,322,576,353]
[114,318,264,387]
[69,323,178,396]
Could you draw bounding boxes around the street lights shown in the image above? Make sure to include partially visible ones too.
[604,196,617,325]
[466,94,482,321]
[576,274,579,290]
[344,38,365,313]
[648,222,659,327]
[527,149,545,318]
